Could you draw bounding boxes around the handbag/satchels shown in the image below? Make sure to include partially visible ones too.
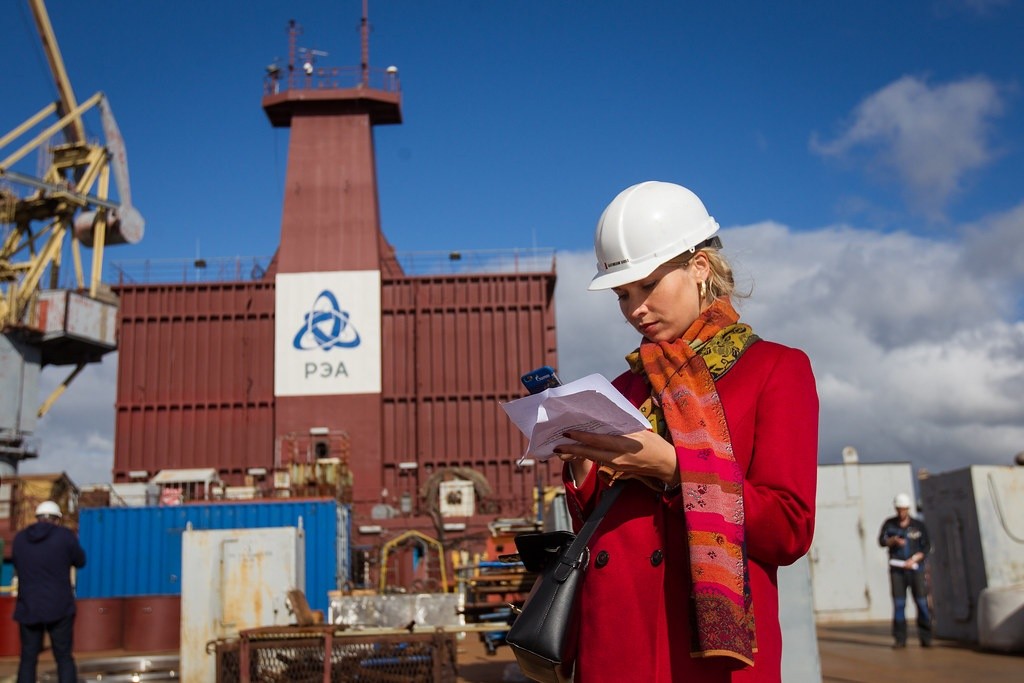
[506,479,634,681]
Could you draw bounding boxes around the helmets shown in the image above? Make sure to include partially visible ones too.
[36,501,62,520]
[893,493,910,509]
[589,179,720,291]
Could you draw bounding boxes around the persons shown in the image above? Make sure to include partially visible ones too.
[12,500,87,683]
[507,182,819,682]
[878,493,933,649]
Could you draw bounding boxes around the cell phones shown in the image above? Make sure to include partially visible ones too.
[521,365,563,395]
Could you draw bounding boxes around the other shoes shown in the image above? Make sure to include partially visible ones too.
[892,640,905,647]
[921,641,931,647]
